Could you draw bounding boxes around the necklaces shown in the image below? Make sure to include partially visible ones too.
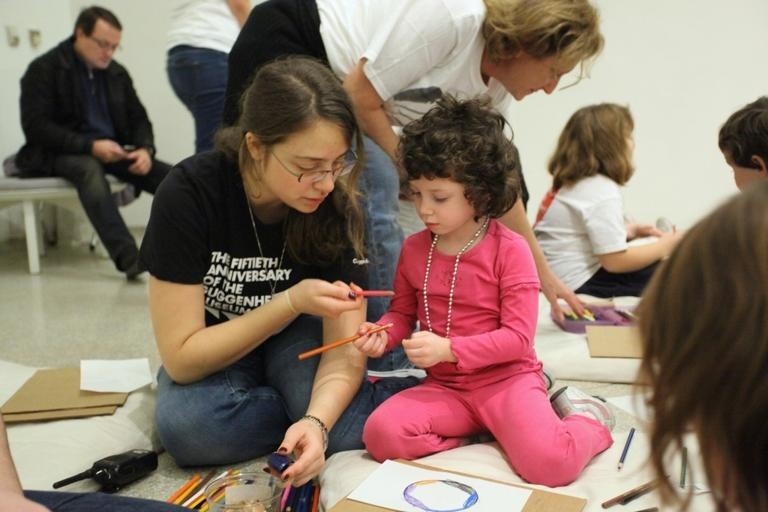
[422,215,489,338]
[246,175,293,303]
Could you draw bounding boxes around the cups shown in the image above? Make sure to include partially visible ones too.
[205,472,284,511]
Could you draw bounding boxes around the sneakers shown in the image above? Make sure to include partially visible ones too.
[365,367,428,382]
[552,386,616,434]
[542,367,557,390]
[125,254,150,280]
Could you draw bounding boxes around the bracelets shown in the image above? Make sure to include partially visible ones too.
[283,287,301,316]
[305,408,336,456]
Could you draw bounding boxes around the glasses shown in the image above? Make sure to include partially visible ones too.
[261,141,360,184]
[88,36,123,54]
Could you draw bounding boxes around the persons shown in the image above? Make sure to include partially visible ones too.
[353,98,620,489]
[0,402,207,511]
[166,0,247,157]
[632,171,767,509]
[13,7,174,284]
[528,104,678,298]
[141,58,417,493]
[222,0,606,371]
[720,97,767,192]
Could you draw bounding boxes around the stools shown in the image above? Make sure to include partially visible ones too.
[0,160,139,274]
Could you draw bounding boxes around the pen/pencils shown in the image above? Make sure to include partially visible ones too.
[351,290,396,297]
[618,428,635,469]
[165,467,321,512]
[297,324,393,360]
[681,447,687,488]
[602,475,670,508]
[562,308,603,321]
